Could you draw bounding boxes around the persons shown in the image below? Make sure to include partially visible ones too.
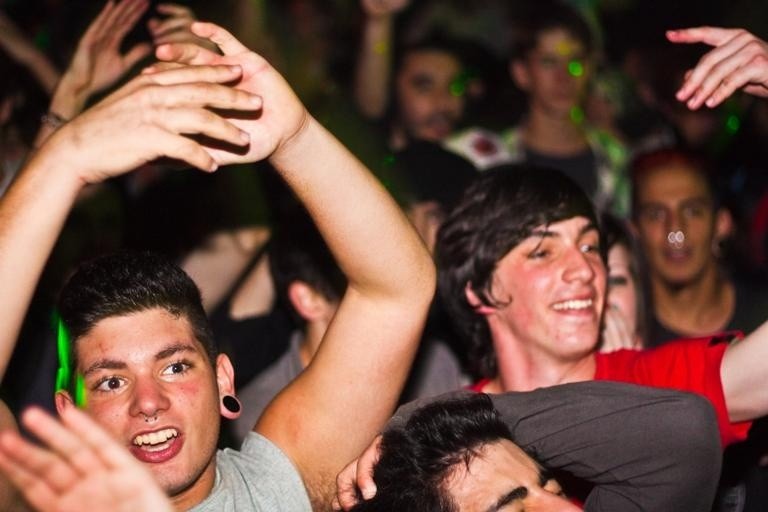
[1,0,768,512]
